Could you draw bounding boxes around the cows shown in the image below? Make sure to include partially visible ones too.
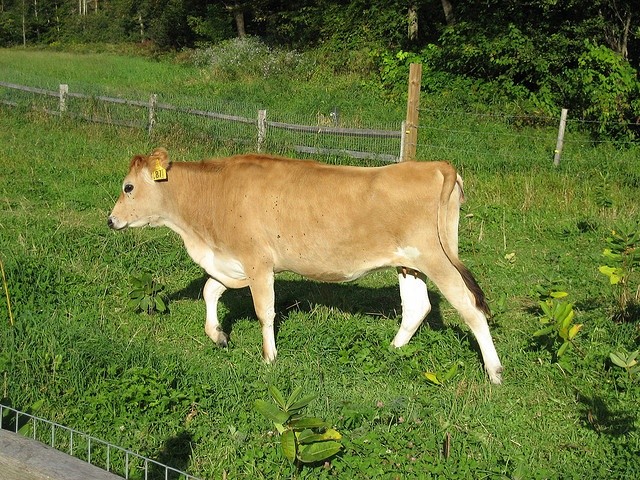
[107,146,504,386]
